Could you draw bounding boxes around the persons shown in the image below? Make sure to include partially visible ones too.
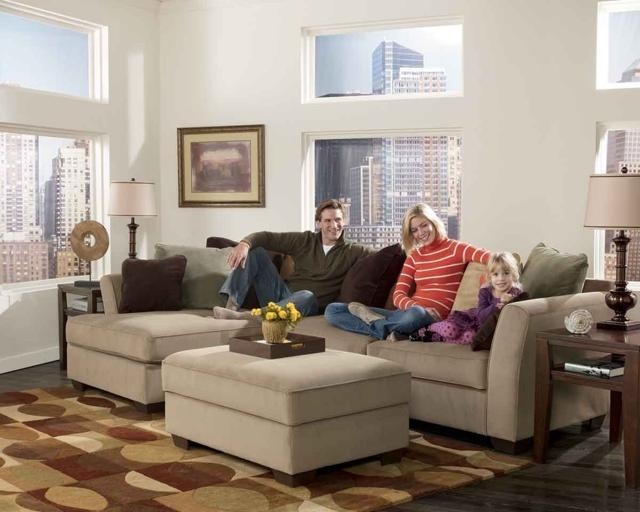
[408,248,522,342]
[323,202,496,344]
[213,197,379,321]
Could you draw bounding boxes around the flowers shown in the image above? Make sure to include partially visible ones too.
[250,297,302,324]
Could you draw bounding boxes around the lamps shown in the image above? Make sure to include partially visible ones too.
[582,165,640,334]
[106,176,160,261]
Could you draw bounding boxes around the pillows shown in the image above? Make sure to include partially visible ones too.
[447,261,491,318]
[338,239,407,308]
[279,252,295,281]
[205,236,286,310]
[519,242,588,300]
[153,239,237,309]
[117,253,188,314]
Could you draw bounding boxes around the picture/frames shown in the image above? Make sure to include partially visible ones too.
[175,123,266,210]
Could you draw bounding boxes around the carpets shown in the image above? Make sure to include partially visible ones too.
[0,383,535,510]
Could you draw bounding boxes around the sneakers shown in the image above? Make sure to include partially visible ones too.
[386,331,398,342]
[348,301,386,325]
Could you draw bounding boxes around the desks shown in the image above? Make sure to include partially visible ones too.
[56,282,106,372]
[530,323,640,490]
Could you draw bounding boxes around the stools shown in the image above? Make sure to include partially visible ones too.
[156,337,417,485]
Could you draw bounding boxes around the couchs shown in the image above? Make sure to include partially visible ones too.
[61,240,624,455]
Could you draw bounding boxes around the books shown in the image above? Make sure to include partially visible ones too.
[564,357,625,379]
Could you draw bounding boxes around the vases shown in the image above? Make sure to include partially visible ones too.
[261,318,290,344]
